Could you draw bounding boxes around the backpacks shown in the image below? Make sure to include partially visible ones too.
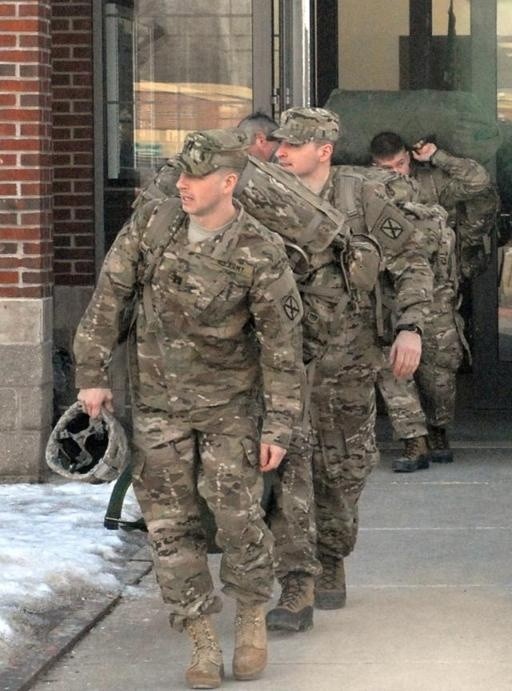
[234,151,345,270]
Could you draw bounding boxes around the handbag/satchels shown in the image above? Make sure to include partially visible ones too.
[457,182,502,280]
[108,278,138,436]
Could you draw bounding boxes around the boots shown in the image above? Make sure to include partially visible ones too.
[232,600,268,680]
[183,613,225,688]
[392,436,429,472]
[266,571,316,632]
[315,553,345,608]
[426,423,453,463]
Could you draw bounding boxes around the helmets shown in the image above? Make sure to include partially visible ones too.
[464,189,501,233]
[45,401,129,485]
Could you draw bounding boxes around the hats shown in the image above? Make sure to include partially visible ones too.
[270,106,340,145]
[166,129,250,177]
